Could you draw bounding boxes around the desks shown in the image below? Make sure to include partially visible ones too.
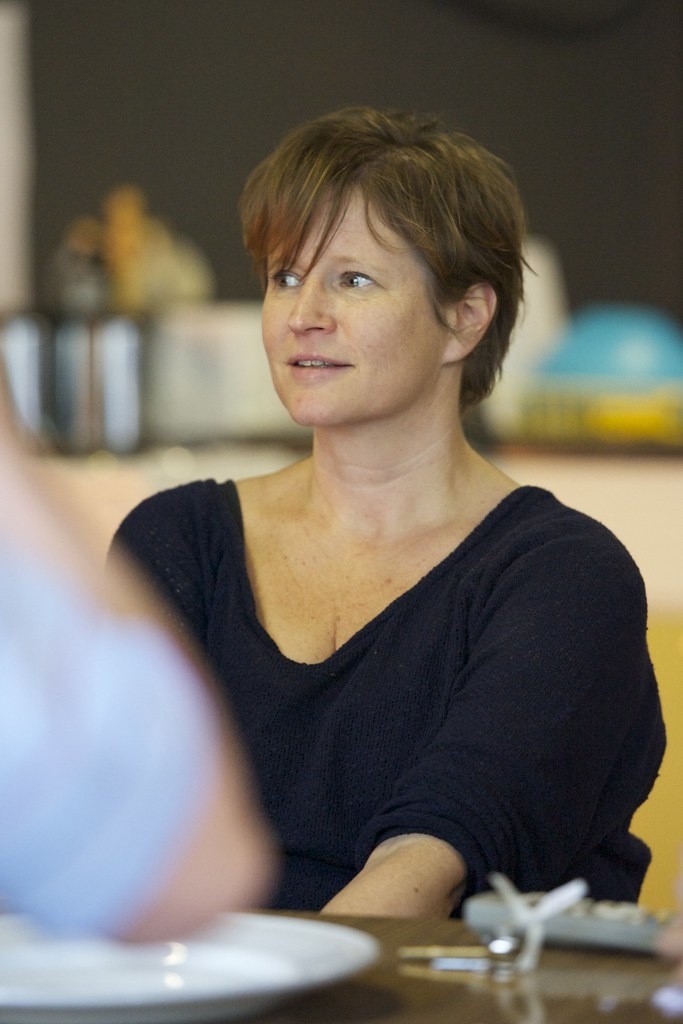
[233,908,683,1024]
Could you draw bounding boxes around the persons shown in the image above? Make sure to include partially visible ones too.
[103,105,662,920]
[0,381,275,944]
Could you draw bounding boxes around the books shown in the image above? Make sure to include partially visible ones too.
[0,309,149,458]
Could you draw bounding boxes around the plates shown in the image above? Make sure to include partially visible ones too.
[0,909,382,1024]
